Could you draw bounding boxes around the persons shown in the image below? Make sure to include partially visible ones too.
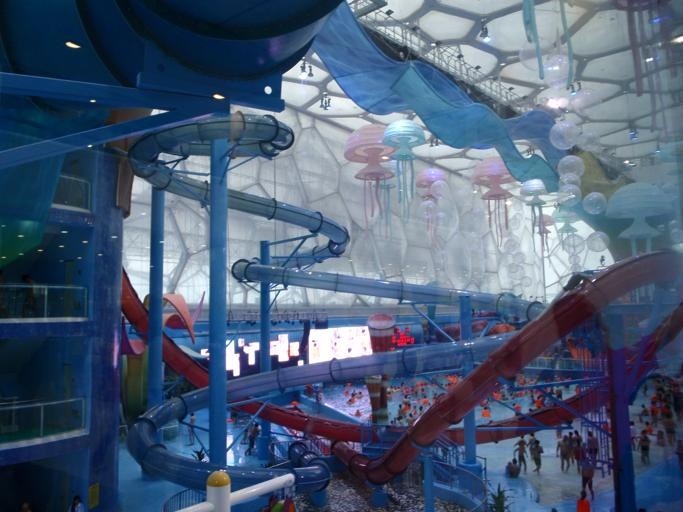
[260,495,279,512]
[576,489,590,512]
[244,369,681,478]
[581,456,595,500]
[19,500,32,512]
[68,496,85,512]
[187,413,196,445]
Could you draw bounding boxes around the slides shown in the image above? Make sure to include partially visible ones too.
[118,110,683,494]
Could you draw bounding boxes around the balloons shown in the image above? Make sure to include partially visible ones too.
[502,32,609,293]
[420,180,453,224]
[658,218,683,253]
